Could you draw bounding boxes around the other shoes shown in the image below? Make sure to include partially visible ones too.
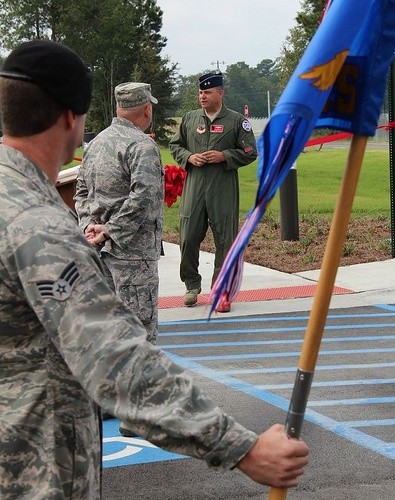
[183,287,202,305]
[217,296,231,312]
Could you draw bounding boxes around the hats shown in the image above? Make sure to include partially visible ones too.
[115,81,158,108]
[199,72,223,90]
[0,40,94,115]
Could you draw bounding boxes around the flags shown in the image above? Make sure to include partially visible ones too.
[202,0,395,322]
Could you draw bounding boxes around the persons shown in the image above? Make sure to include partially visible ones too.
[168,69,259,312]
[72,82,165,439]
[0,39,309,500]
